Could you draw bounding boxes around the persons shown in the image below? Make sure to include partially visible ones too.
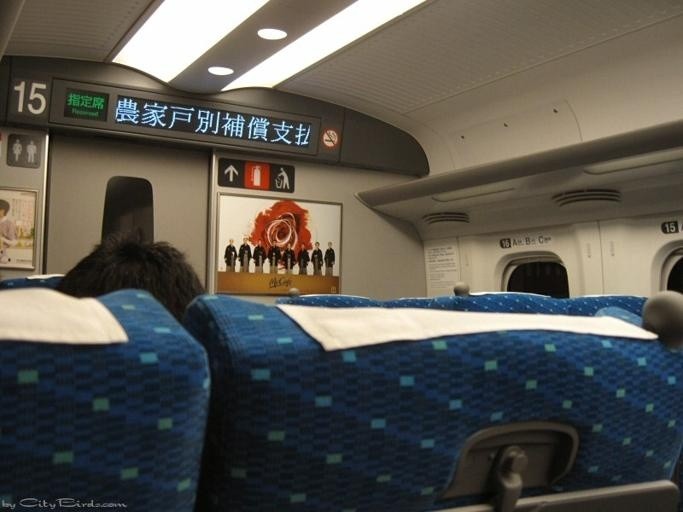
[267,240,281,273]
[298,242,310,275]
[0,201,17,267]
[253,239,267,274]
[223,239,237,272]
[282,241,297,274]
[54,225,209,322]
[325,242,335,276]
[238,237,252,273]
[311,242,324,275]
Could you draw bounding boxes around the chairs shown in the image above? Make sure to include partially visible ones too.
[0,273,682,512]
